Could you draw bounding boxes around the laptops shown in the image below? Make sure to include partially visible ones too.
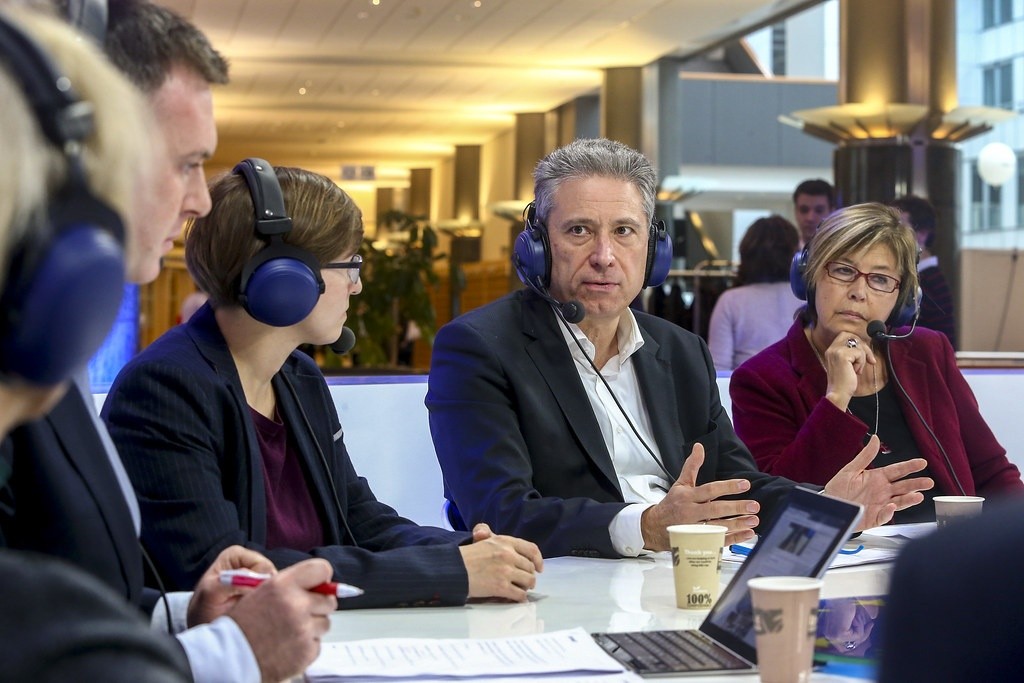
[590,486,864,681]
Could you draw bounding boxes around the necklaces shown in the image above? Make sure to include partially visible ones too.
[809,328,894,456]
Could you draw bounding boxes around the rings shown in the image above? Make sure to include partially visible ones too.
[702,521,707,525]
[847,338,858,348]
[424,133,936,564]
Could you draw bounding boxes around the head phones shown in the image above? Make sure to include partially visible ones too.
[514,199,673,289]
[790,211,923,328]
[0,13,128,386]
[233,158,325,328]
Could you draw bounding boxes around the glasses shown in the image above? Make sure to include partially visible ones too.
[824,261,902,294]
[316,252,363,284]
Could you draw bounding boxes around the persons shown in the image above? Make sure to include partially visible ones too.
[892,196,954,352]
[705,214,815,375]
[867,494,1024,683]
[728,203,1024,526]
[0,0,337,683]
[790,179,837,251]
[98,157,544,609]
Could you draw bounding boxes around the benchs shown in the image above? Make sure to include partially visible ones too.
[322,368,1024,532]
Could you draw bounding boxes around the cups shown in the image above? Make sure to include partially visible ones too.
[666,523,728,610]
[749,576,824,683]
[933,495,985,527]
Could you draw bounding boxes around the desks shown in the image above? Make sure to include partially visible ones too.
[668,270,737,341]
[287,518,1024,682]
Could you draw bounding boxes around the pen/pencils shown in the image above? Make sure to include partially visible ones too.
[217,570,365,598]
[848,338,858,348]
[729,544,752,556]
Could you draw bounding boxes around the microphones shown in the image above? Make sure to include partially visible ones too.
[510,251,585,324]
[330,326,356,354]
[867,306,921,340]
